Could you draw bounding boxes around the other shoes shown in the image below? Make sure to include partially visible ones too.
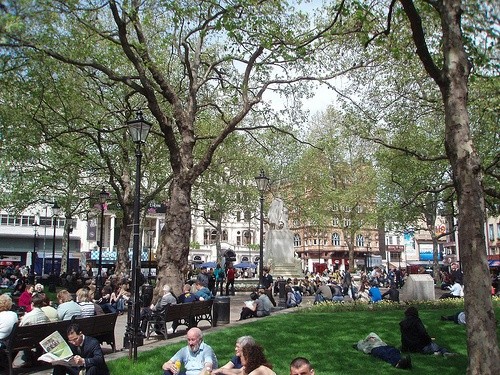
[444,352,459,358]
[434,351,442,356]
[400,354,412,370]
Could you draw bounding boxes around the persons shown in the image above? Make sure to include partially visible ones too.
[441,311,466,324]
[290,357,314,375]
[52,324,109,375]
[0,263,142,363]
[274,265,404,308]
[239,289,275,320]
[429,269,500,299]
[399,307,458,357]
[138,280,213,336]
[162,327,218,375]
[263,267,276,307]
[197,263,236,296]
[357,332,412,370]
[210,335,276,375]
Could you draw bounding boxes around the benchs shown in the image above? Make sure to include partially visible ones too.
[144,297,216,340]
[0,313,118,375]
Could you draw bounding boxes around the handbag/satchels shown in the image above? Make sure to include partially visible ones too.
[217,268,225,278]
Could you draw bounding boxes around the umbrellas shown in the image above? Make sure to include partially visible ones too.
[199,262,217,267]
[234,262,256,268]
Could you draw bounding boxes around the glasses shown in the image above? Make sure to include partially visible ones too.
[67,335,80,343]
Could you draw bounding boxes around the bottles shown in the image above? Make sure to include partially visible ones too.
[172,359,181,375]
[204,367,211,375]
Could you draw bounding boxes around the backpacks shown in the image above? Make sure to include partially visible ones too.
[291,292,302,304]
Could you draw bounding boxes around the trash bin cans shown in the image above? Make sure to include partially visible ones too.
[212,296,231,328]
[141,284,153,307]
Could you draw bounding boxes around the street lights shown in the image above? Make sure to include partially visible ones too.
[253,168,269,294]
[95,186,110,300]
[48,200,61,292]
[125,109,152,350]
[30,221,40,285]
[65,213,73,275]
